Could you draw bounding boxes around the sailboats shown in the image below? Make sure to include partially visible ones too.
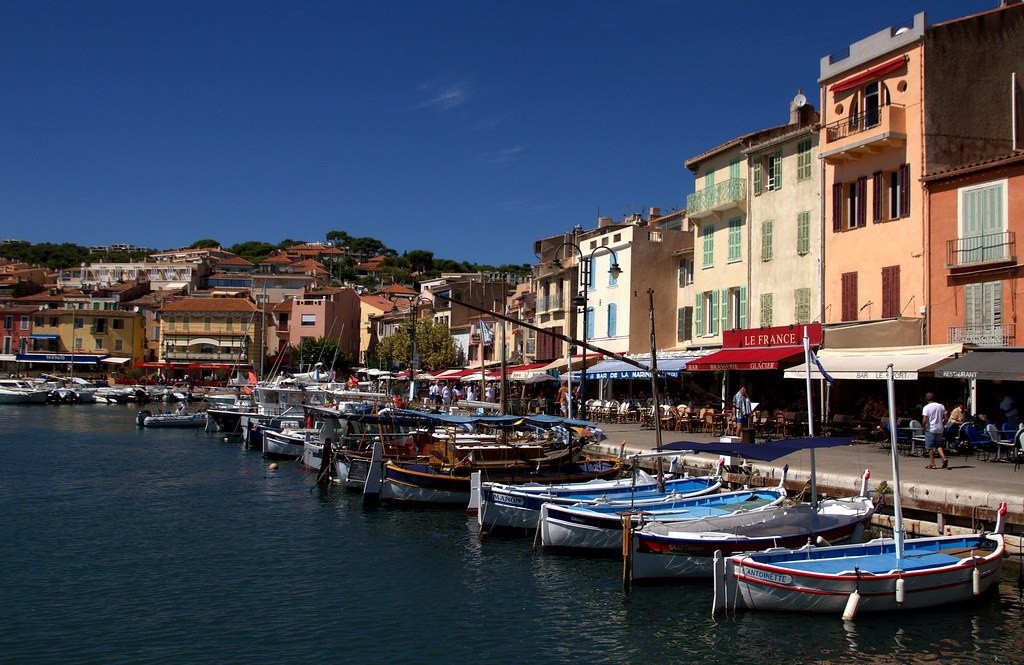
[531,286,789,559]
[620,323,888,589]
[710,364,1009,616]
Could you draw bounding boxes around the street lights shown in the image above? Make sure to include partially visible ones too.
[392,296,436,411]
[550,242,624,445]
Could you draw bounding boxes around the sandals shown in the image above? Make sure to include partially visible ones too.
[925,464,936,469]
[942,458,948,468]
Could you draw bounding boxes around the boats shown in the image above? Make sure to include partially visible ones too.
[0,283,628,514]
[466,449,674,545]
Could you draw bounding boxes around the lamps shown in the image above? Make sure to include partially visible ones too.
[760,326,763,331]
[789,324,793,330]
[731,329,735,333]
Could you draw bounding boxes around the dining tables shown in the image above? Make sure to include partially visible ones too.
[988,429,1017,460]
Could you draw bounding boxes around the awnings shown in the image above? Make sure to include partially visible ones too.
[135,361,254,371]
[0,353,132,365]
[396,346,1024,378]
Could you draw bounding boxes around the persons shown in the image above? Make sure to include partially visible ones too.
[361,375,582,419]
[857,392,990,469]
[5,367,127,386]
[996,391,1020,425]
[249,383,581,464]
[142,372,228,403]
[732,385,752,437]
[622,392,713,431]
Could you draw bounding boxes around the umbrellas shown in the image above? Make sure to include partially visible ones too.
[352,365,397,380]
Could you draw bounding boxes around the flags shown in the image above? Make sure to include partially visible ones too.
[480,318,492,346]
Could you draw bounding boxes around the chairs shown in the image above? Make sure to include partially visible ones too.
[585,398,1024,472]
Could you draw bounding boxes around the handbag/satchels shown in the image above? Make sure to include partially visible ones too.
[562,387,573,401]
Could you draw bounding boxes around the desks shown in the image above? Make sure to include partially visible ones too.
[898,427,925,457]
